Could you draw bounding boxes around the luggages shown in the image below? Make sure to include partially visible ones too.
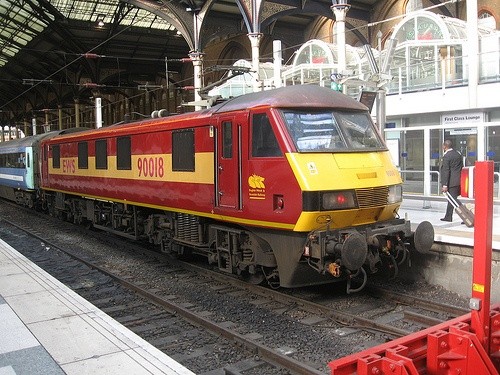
[443,191,474,228]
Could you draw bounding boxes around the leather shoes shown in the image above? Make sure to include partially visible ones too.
[440,216,452,222]
[461,222,465,224]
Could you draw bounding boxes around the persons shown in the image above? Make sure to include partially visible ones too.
[440,140,463,222]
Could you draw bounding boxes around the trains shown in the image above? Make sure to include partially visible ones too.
[0,84,435,294]
